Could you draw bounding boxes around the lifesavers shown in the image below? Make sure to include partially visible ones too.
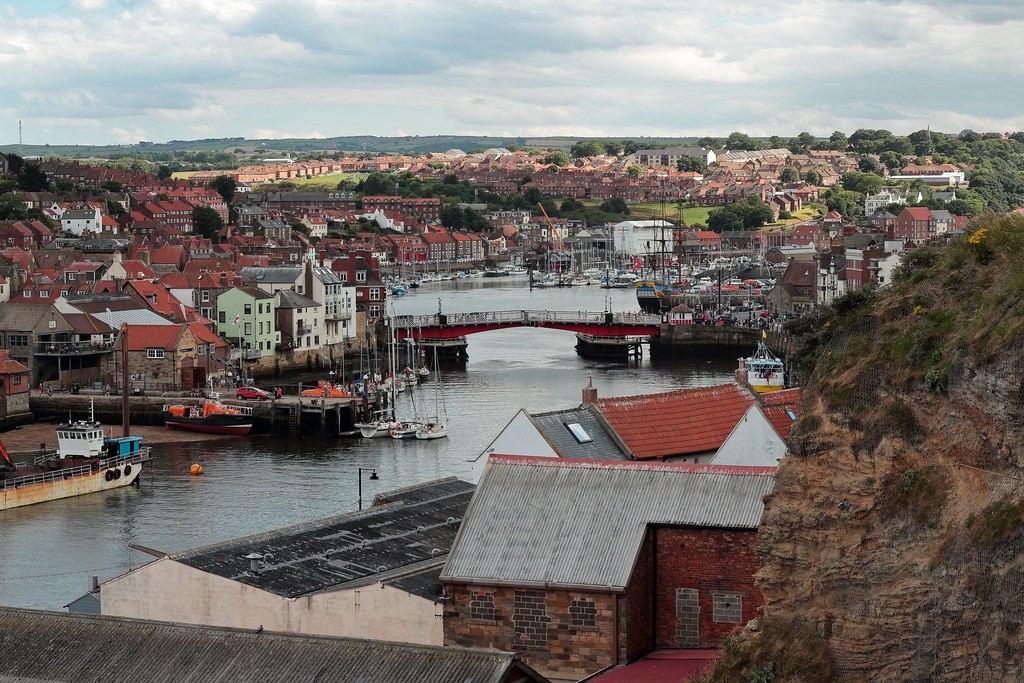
[105,470,114,481]
[113,469,121,480]
[124,465,132,475]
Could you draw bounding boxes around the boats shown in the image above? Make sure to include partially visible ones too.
[0,397,155,512]
[353,421,397,438]
[743,327,785,391]
[575,294,643,360]
[381,243,527,294]
[163,374,253,436]
[415,297,469,363]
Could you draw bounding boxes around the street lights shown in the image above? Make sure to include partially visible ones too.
[359,467,379,510]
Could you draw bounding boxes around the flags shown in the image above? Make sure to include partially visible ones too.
[232,314,239,324]
[762,330,766,339]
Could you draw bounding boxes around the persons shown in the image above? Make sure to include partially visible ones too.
[732,312,774,329]
[341,384,349,394]
[595,312,604,321]
[135,387,139,392]
[784,367,798,385]
[48,384,52,394]
[38,383,43,395]
[45,342,107,352]
[105,384,111,397]
[298,398,302,404]
[60,383,65,396]
[323,382,328,395]
[277,387,282,401]
[310,395,318,405]
[288,340,298,347]
[273,386,278,399]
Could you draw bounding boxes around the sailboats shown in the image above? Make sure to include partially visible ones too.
[528,222,789,289]
[636,177,692,313]
[415,343,451,440]
[331,300,430,407]
[390,345,425,438]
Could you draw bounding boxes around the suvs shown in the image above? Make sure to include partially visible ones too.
[236,386,271,401]
